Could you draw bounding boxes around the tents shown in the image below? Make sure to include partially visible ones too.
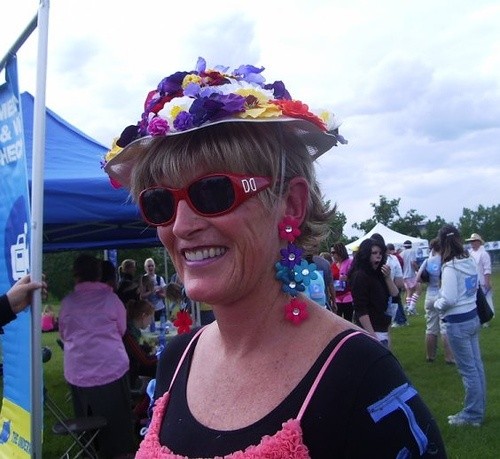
[344,223,429,263]
[18,92,202,330]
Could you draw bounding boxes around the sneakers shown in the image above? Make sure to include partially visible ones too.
[445,358,456,365]
[447,414,482,426]
[391,321,410,328]
[425,355,435,361]
[405,307,420,316]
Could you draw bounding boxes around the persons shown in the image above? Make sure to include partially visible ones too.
[304,223,423,344]
[412,236,456,364]
[59,255,196,454]
[467,233,496,327]
[0,273,49,333]
[431,223,488,427]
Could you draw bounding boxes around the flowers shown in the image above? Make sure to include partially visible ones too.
[101,56,347,189]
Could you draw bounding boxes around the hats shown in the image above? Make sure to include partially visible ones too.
[402,240,412,246]
[101,57,347,186]
[464,233,485,244]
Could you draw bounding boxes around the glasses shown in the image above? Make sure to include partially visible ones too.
[139,172,280,226]
[330,251,336,254]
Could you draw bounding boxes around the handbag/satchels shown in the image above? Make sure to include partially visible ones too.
[477,285,494,323]
[420,260,430,283]
